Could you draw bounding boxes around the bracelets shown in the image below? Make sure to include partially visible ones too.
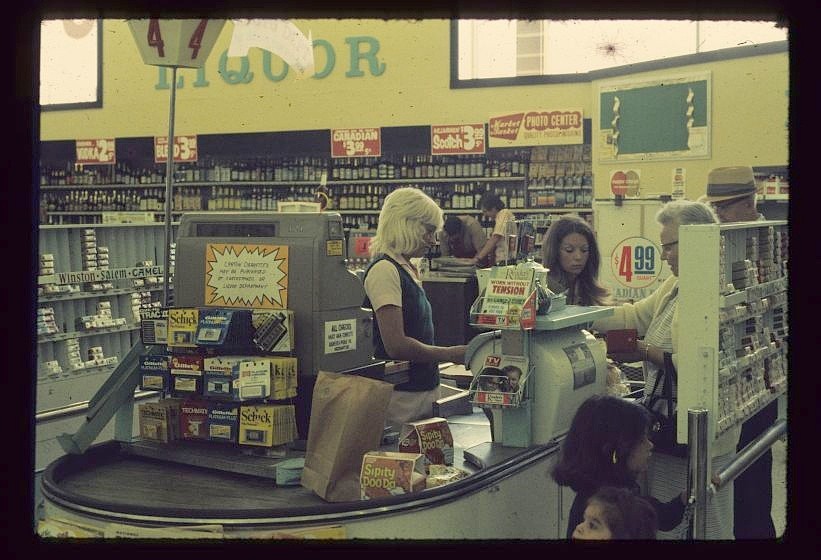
[476,256,480,261]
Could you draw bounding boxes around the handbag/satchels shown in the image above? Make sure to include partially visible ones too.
[639,351,688,459]
[301,369,394,504]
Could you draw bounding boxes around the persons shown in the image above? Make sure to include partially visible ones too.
[697,165,778,538]
[540,214,606,306]
[481,366,522,393]
[360,187,469,432]
[572,486,658,539]
[473,193,518,266]
[440,216,487,258]
[551,395,688,538]
[587,201,743,539]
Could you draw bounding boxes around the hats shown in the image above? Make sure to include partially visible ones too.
[696,166,764,203]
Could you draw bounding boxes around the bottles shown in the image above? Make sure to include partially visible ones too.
[40,148,529,274]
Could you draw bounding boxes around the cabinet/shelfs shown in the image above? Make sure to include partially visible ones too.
[674,218,790,540]
[38,153,592,488]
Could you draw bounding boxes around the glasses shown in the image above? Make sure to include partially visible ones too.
[709,198,745,214]
[661,240,679,254]
